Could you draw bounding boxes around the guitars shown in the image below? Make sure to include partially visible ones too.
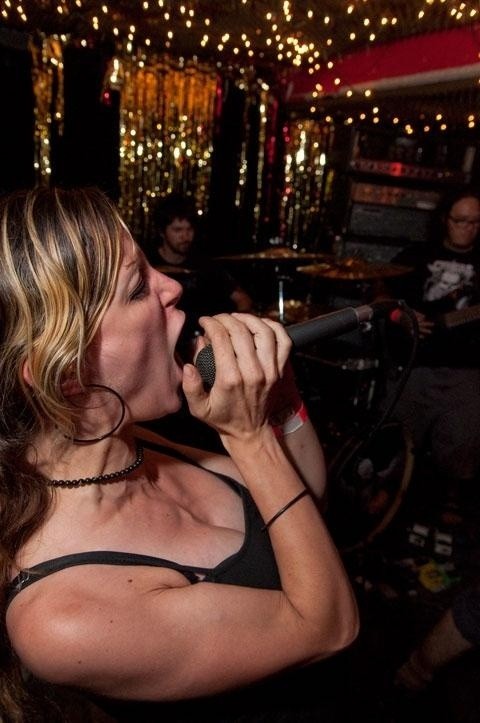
[406,286,480,352]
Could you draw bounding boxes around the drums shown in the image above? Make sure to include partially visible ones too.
[299,389,414,557]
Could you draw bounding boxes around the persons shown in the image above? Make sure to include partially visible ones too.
[0,178,362,722]
[145,191,255,314]
[365,185,480,528]
[388,574,480,696]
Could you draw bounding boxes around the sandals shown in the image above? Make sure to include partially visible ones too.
[435,491,465,535]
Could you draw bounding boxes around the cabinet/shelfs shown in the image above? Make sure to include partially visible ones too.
[321,126,480,310]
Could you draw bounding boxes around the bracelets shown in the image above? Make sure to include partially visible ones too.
[258,486,312,535]
[272,399,309,438]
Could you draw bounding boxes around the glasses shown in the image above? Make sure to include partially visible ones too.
[448,213,480,228]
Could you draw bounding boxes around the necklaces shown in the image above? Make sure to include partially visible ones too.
[43,434,147,487]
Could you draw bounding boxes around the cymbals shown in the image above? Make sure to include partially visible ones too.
[296,259,412,279]
[215,252,329,261]
[151,264,190,273]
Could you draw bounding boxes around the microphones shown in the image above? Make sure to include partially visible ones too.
[193,306,373,391]
